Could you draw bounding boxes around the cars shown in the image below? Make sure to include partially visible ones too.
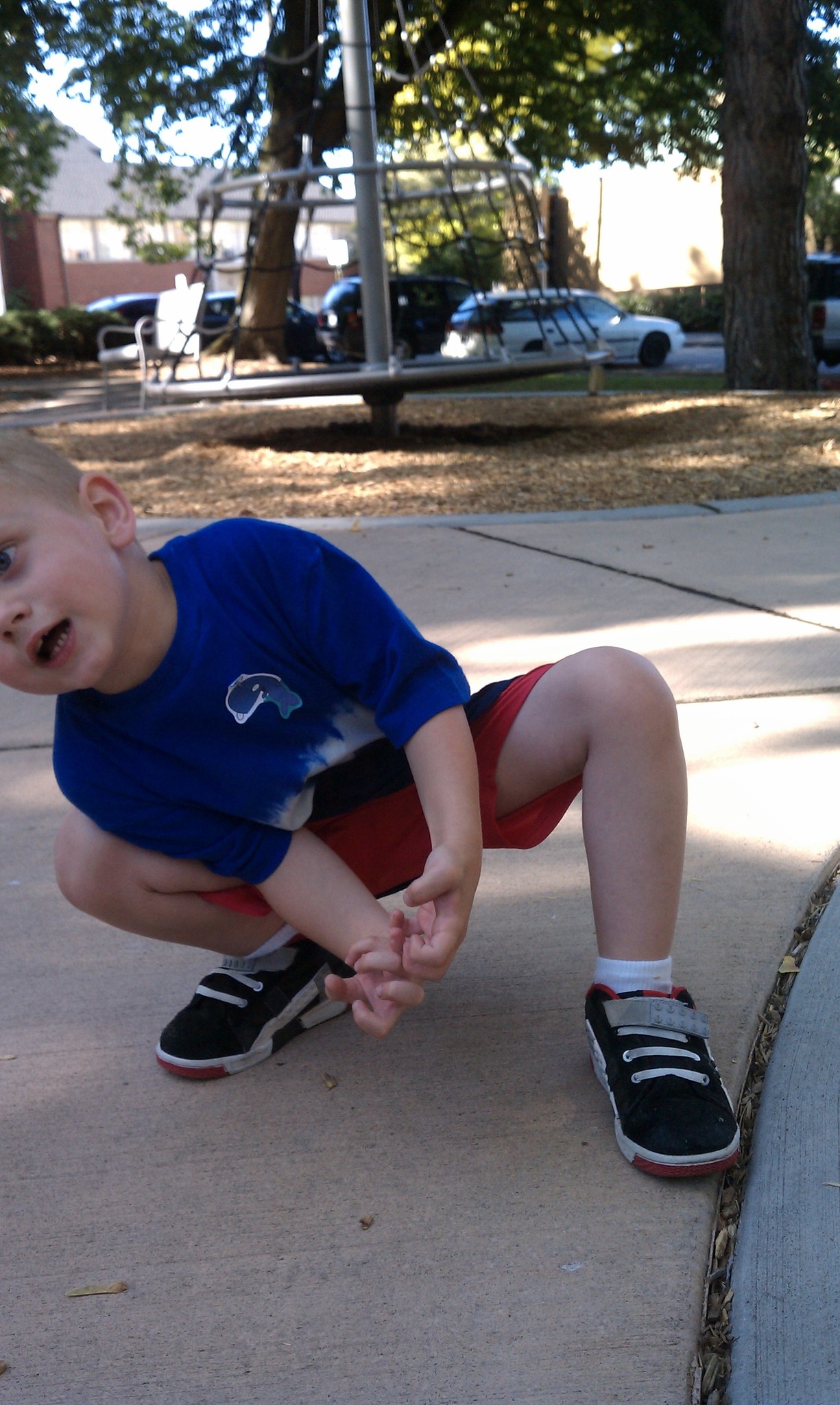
[81,293,167,344]
[439,287,687,371]
[190,288,327,367]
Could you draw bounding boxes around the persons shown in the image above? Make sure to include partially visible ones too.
[0,427,743,1177]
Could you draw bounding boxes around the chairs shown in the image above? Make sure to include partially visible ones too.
[98,282,207,410]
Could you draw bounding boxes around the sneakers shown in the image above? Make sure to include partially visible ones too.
[582,981,741,1178]
[157,932,355,1081]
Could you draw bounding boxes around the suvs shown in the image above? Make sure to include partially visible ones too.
[800,253,839,366]
[314,275,483,355]
